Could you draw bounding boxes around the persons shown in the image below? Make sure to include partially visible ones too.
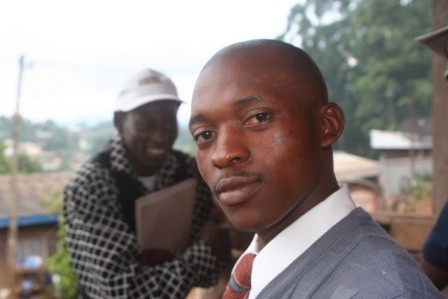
[185,39,446,298]
[60,67,224,299]
[414,20,448,291]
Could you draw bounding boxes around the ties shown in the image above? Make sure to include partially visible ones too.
[221,252,256,299]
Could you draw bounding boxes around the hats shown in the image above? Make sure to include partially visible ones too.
[113,67,187,113]
